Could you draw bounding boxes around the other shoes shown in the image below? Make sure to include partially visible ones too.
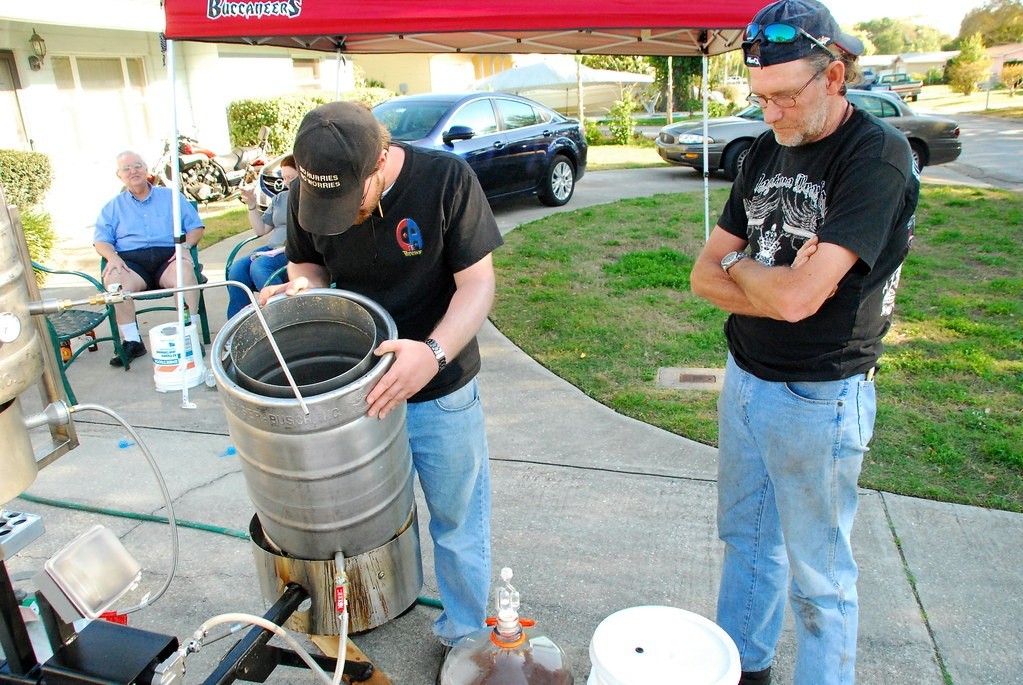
[738,668,772,685]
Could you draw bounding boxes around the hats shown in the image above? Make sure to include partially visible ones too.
[290,99,383,236]
[741,0,865,67]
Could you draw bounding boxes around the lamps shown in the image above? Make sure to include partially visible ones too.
[28,27,46,72]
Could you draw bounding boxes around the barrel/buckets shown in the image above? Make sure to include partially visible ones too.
[149,322,207,393]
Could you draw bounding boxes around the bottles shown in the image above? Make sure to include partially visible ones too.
[60,340,72,363]
[85,329,98,351]
[183,297,191,326]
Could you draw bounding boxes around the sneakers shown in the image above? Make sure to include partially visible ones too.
[109,334,147,367]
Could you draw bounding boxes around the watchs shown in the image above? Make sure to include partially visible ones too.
[720,251,748,274]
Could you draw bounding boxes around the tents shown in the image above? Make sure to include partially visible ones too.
[162,0,779,408]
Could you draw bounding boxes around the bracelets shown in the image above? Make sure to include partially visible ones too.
[424,339,447,373]
[249,204,256,210]
[183,243,191,249]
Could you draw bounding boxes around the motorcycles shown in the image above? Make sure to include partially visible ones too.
[149,127,272,206]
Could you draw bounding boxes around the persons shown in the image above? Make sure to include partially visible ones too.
[692,0,918,685]
[224,154,299,351]
[259,103,503,685]
[92,150,204,366]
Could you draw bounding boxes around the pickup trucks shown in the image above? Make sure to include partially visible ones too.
[870,72,924,101]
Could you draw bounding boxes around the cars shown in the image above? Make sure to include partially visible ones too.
[728,75,742,84]
[259,92,587,220]
[653,89,963,184]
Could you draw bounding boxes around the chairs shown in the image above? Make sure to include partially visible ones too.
[422,110,441,129]
[25,200,289,409]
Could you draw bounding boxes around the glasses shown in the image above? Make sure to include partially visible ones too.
[359,175,373,207]
[117,162,143,171]
[745,68,824,109]
[742,22,836,60]
